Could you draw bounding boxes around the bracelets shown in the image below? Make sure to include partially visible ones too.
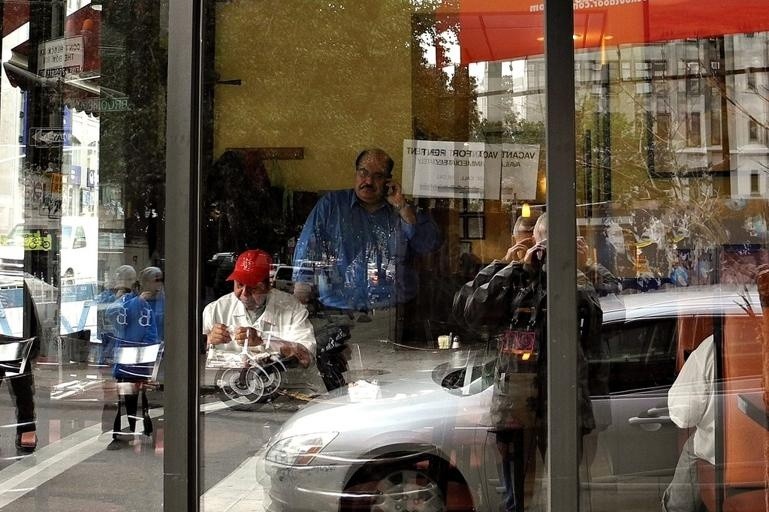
[392,199,408,216]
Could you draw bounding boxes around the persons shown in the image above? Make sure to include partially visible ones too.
[291,148,444,397]
[451,210,623,511]
[107,266,166,449]
[464,211,605,511]
[88,264,155,445]
[202,249,329,426]
[658,331,716,511]
[1,237,46,455]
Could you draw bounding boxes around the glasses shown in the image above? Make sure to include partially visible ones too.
[357,167,393,181]
[154,278,163,283]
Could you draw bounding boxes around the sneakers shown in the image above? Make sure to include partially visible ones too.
[107,437,129,450]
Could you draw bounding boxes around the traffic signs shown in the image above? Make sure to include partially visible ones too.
[29,126,81,151]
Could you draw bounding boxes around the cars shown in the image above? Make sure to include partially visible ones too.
[0,268,106,378]
[207,250,396,310]
[256,282,767,510]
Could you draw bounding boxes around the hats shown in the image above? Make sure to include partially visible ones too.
[113,264,137,290]
[138,266,163,291]
[226,248,272,290]
